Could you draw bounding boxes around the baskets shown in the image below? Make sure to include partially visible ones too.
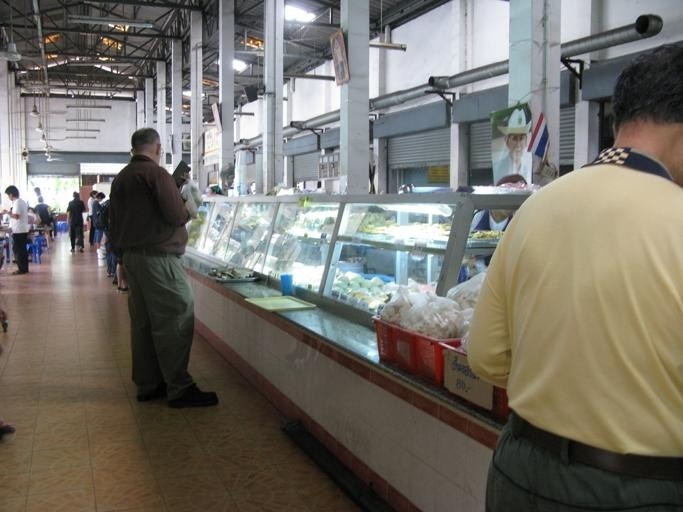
[370,311,510,419]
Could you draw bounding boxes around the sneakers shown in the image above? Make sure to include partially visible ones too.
[135,383,170,402]
[168,383,219,409]
[10,258,26,275]
[106,271,129,295]
[69,243,102,254]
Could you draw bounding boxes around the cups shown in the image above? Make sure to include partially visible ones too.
[280,272,295,296]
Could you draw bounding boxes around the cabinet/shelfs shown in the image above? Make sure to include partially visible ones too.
[185,192,534,334]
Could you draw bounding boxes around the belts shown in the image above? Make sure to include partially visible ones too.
[507,408,682,481]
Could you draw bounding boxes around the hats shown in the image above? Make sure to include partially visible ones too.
[495,108,532,135]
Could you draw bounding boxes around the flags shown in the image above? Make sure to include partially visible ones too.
[525,113,549,158]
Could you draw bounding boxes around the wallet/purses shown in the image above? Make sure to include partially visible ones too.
[171,160,191,189]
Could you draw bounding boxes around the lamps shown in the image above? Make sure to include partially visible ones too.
[2,1,54,162]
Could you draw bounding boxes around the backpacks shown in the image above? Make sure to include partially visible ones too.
[95,204,109,227]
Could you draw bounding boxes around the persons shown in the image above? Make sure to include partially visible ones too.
[0,185,32,275]
[32,197,58,241]
[454,174,534,287]
[85,189,129,293]
[65,191,85,252]
[493,108,534,183]
[109,127,219,409]
[26,207,41,226]
[459,37,681,511]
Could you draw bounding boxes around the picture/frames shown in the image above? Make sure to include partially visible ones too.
[328,27,350,86]
